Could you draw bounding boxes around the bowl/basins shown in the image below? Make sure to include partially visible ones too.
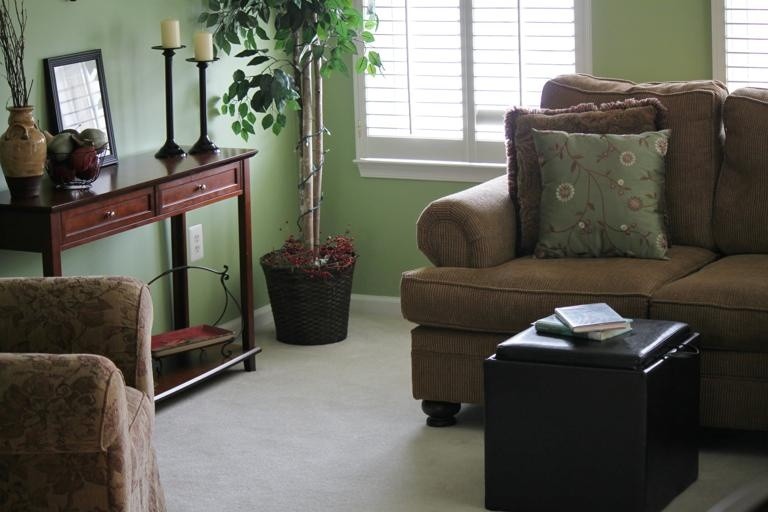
[45,152,107,191]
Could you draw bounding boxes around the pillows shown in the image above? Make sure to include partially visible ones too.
[505,97,673,259]
[530,127,674,262]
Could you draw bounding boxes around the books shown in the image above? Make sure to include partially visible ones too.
[534,314,634,342]
[554,301,627,333]
[151,323,236,359]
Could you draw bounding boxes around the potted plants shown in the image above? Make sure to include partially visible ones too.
[1,0,47,199]
[196,0,386,346]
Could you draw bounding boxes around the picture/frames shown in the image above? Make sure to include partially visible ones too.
[41,49,120,168]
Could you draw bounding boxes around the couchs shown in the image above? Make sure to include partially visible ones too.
[0,274,169,512]
[399,73,768,428]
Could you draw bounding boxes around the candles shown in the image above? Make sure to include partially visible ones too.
[160,18,181,48]
[192,32,214,61]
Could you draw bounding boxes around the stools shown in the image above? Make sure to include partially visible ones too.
[483,318,703,512]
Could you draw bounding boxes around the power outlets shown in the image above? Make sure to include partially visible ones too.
[187,223,205,261]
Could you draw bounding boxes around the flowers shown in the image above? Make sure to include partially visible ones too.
[262,223,361,282]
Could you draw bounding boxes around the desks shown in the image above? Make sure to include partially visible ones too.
[0,145,263,402]
